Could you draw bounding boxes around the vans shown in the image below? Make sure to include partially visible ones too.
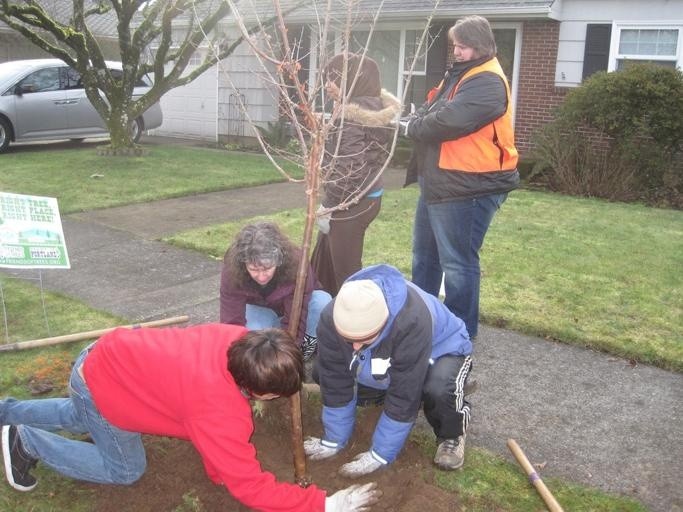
[0,56,162,153]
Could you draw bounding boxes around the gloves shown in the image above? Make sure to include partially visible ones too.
[315,207,330,234]
[323,482,383,512]
[336,449,388,478]
[303,438,341,460]
[390,118,412,135]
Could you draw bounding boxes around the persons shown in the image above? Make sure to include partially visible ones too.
[217,221,333,392]
[300,262,475,478]
[396,13,521,344]
[0,323,384,512]
[309,52,403,300]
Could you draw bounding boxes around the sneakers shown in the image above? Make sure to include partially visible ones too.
[1,424,37,492]
[432,436,466,469]
